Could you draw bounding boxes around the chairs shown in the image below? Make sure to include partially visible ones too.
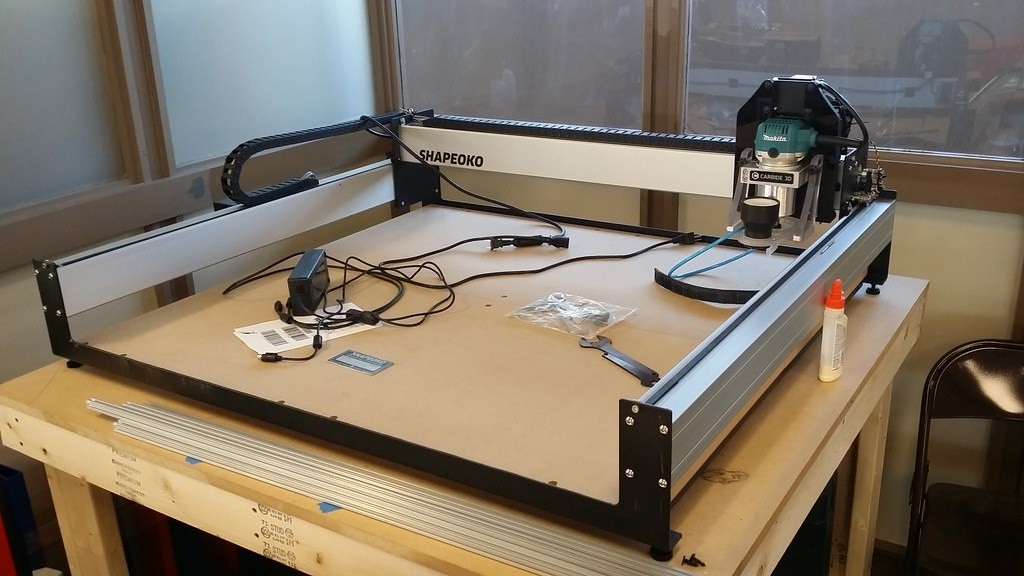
[907,339,1024,576]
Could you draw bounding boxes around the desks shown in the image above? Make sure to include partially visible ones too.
[0,201,930,576]
